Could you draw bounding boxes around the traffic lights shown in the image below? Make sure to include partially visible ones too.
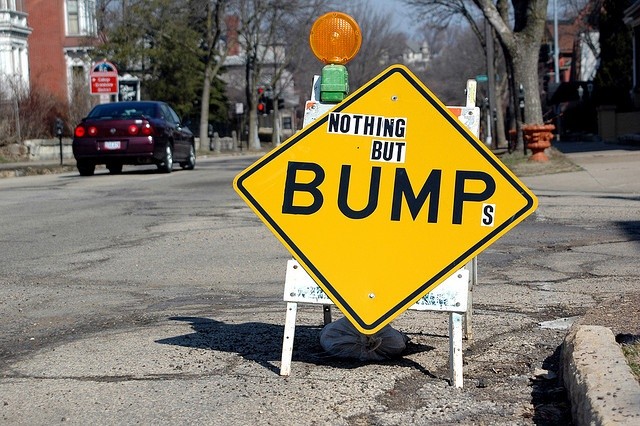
[257,87,264,114]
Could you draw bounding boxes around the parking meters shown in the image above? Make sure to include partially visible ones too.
[56,118,63,164]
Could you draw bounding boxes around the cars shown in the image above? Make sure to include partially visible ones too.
[72,100,196,175]
